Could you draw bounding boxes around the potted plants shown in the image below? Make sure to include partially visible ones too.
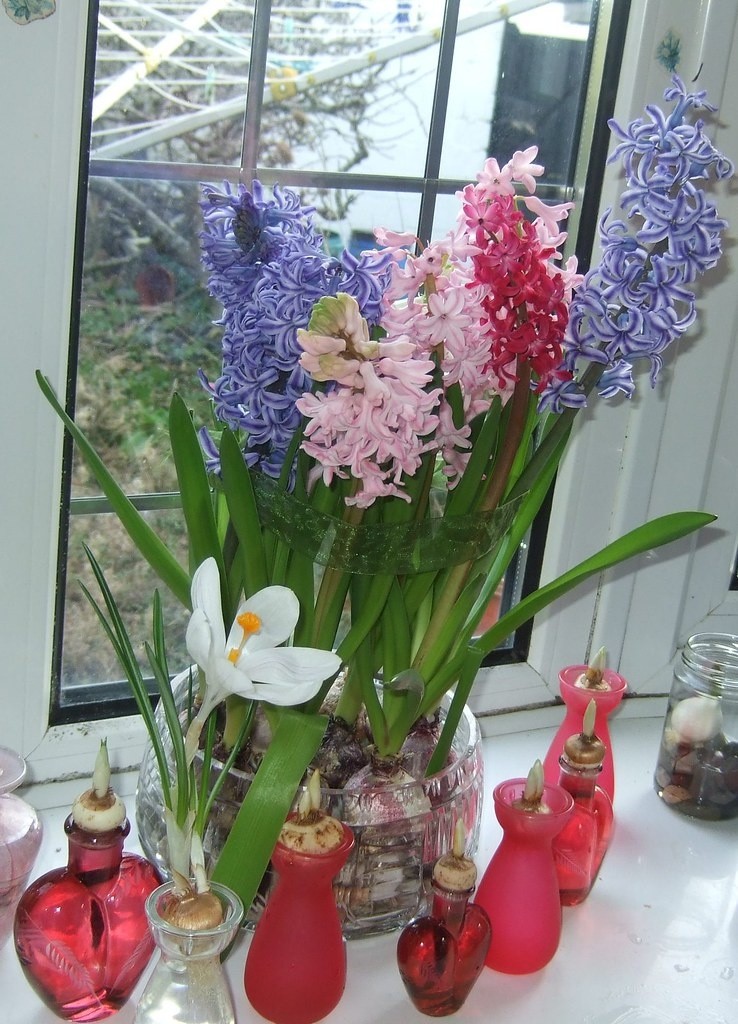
[653,631,738,821]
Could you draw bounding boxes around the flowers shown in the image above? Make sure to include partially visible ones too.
[32,26,738,849]
[70,540,344,929]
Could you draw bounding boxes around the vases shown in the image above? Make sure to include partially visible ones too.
[544,663,629,803]
[134,877,244,1024]
[552,753,614,907]
[134,662,485,942]
[244,811,356,1024]
[397,875,493,1017]
[0,745,44,952]
[473,778,576,976]
[12,816,164,1024]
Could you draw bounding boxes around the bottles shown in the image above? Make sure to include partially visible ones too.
[0,746,43,907]
[554,754,606,906]
[592,783,613,885]
[13,813,163,1024]
[647,632,738,821]
[396,882,492,1018]
[472,778,575,975]
[244,814,356,1024]
[542,665,628,806]
